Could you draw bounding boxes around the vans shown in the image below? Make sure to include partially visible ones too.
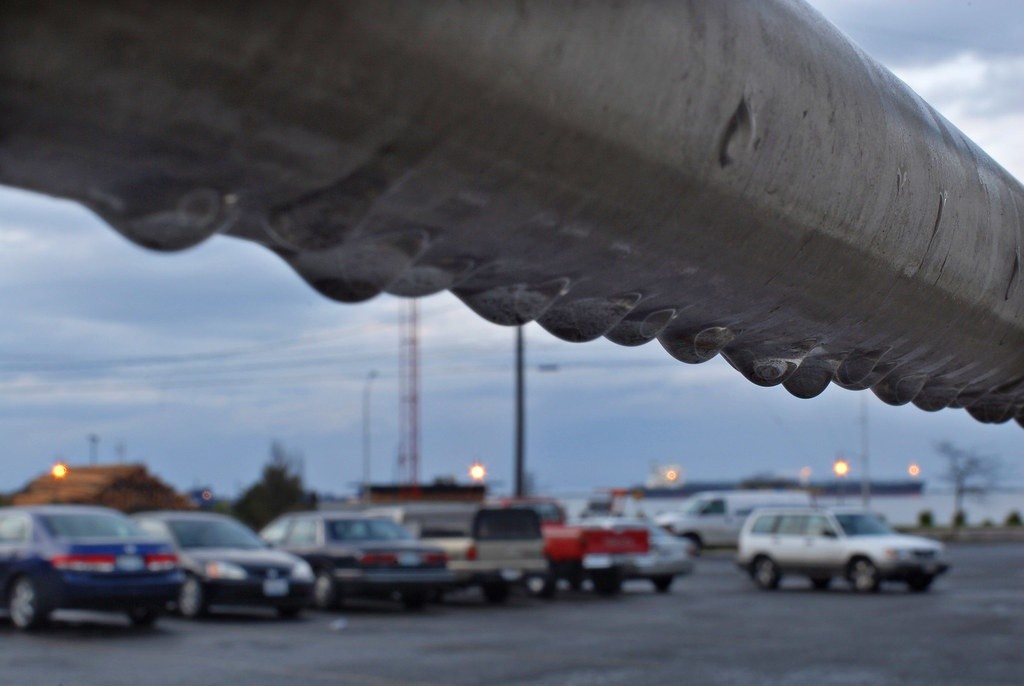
[648,491,812,556]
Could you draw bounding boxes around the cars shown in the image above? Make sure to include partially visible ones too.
[258,509,448,616]
[0,501,186,636]
[574,515,697,593]
[129,510,318,621]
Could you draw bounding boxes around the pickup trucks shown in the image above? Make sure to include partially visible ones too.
[488,493,652,603]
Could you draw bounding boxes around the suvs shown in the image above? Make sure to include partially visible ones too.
[733,504,948,597]
[366,503,552,611]
